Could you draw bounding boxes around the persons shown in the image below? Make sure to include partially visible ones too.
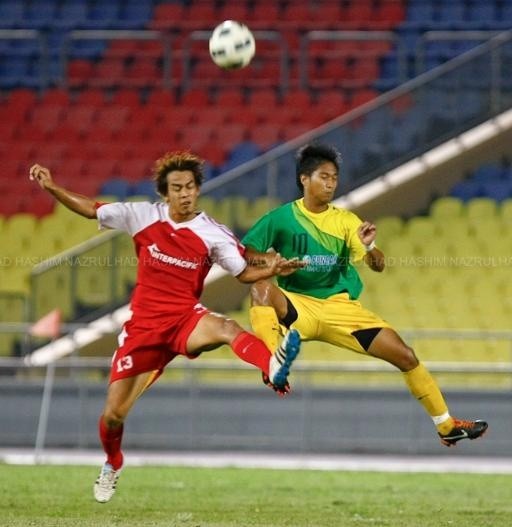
[240,140,487,448]
[27,148,307,504]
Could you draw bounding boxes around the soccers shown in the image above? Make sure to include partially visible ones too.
[209,20,256,72]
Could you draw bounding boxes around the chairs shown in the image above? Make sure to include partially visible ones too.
[0,0,512,383]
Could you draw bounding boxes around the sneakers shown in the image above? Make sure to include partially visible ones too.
[93,451,124,504]
[436,418,489,448]
[261,329,302,396]
